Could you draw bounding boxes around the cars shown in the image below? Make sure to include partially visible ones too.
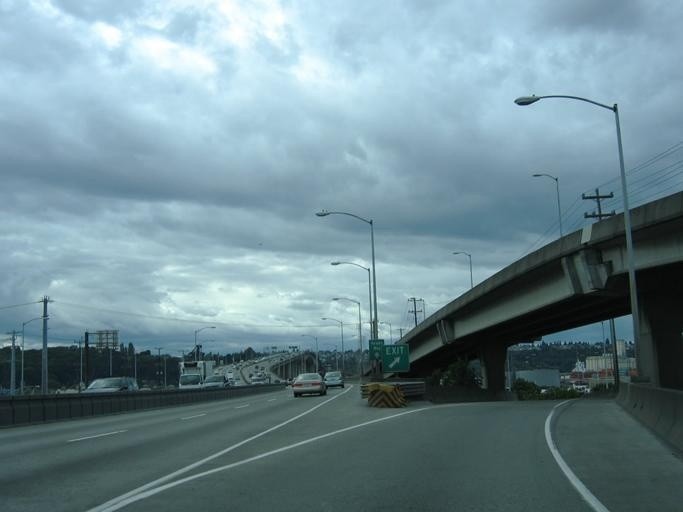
[323,371,344,389]
[200,373,231,389]
[291,373,327,398]
[81,376,139,394]
[214,357,280,386]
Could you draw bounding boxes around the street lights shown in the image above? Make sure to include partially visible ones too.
[315,210,384,383]
[453,251,473,289]
[20,316,50,397]
[194,326,216,361]
[379,321,393,345]
[533,173,563,239]
[517,94,651,383]
[301,334,318,373]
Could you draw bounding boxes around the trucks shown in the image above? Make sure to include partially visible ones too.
[177,360,216,389]
